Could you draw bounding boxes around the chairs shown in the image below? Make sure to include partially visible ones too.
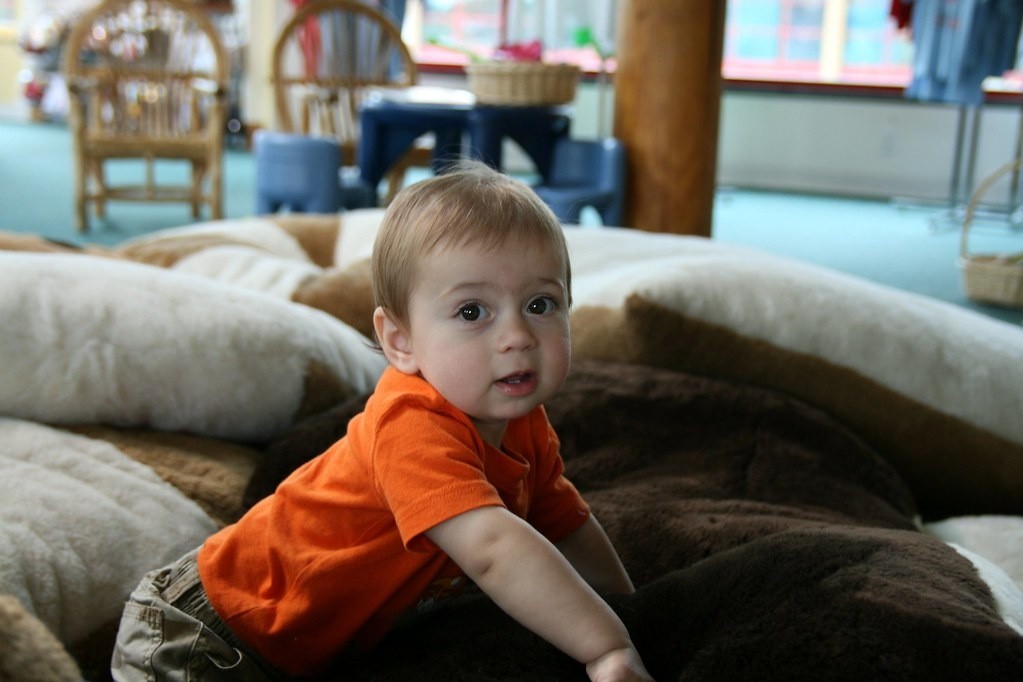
[533,138,630,221]
[271,0,440,206]
[252,135,367,218]
[65,0,230,229]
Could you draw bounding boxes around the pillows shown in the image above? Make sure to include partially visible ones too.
[0,249,389,441]
[553,220,1023,524]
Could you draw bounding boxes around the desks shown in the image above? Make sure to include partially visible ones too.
[357,99,572,204]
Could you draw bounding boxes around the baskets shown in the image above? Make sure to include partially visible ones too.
[464,61,580,106]
[957,159,1023,306]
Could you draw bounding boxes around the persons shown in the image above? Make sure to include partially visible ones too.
[110,154,650,681]
